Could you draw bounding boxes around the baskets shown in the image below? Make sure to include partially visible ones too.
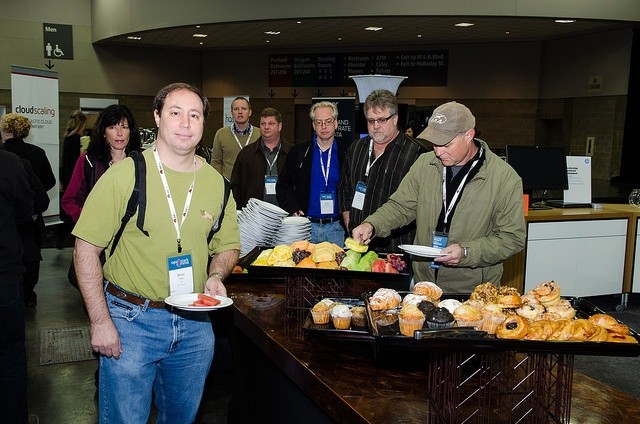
[286,273,388,310]
[425,348,575,424]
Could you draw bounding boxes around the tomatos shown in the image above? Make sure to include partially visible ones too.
[193,293,220,306]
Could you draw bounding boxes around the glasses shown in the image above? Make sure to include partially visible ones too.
[367,113,397,125]
[314,118,336,126]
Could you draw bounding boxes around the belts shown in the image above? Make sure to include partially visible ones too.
[308,217,339,225]
[99,278,165,309]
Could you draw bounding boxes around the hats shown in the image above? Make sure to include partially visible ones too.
[416,101,476,147]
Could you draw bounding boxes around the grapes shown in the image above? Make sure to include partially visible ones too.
[390,253,406,270]
[292,248,312,265]
[335,251,347,265]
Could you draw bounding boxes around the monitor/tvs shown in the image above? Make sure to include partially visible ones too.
[505,144,568,210]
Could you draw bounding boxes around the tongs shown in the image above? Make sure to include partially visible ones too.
[342,235,370,250]
[414,326,488,342]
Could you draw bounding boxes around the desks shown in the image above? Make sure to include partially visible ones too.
[501,203,640,313]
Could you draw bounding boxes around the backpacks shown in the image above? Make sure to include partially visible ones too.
[68,152,233,291]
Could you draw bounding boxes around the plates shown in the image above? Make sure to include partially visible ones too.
[165,293,234,312]
[398,244,449,258]
[237,198,312,257]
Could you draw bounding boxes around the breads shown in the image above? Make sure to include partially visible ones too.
[371,287,401,310]
[454,279,638,343]
[415,280,444,298]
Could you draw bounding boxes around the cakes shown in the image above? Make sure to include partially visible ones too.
[311,297,330,324]
[398,300,435,337]
[330,301,353,329]
[426,306,454,328]
[350,306,367,328]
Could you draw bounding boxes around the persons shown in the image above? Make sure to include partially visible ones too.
[337,89,429,253]
[0,148,35,424]
[70,81,241,424]
[231,107,294,210]
[210,97,261,183]
[352,100,527,293]
[0,111,57,313]
[275,101,345,249]
[61,110,86,195]
[62,104,141,263]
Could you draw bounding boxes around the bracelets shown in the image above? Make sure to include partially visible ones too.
[209,272,224,280]
